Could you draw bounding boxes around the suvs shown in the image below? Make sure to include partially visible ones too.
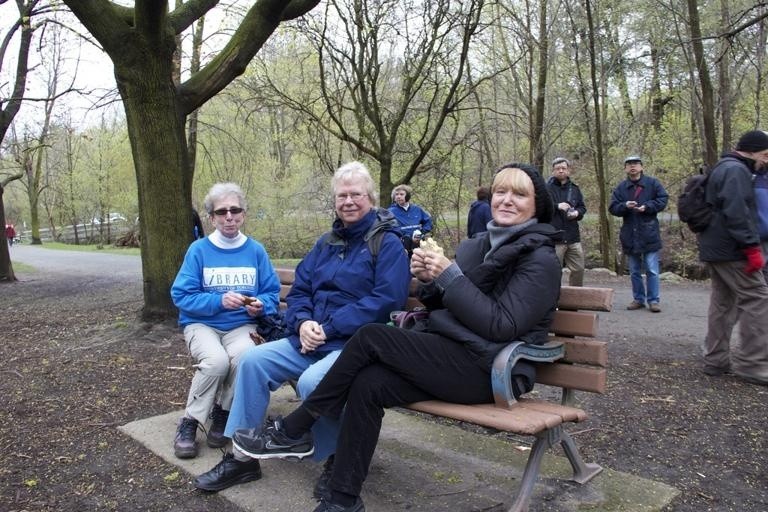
[105,213,121,221]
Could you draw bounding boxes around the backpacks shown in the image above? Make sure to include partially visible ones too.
[679,158,738,233]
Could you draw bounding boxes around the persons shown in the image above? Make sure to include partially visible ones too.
[6,224,16,246]
[191,161,414,501]
[388,184,433,258]
[467,187,493,239]
[170,182,281,458]
[751,163,768,286]
[697,130,768,386]
[192,207,204,240]
[608,156,669,312]
[546,158,587,287]
[232,163,562,512]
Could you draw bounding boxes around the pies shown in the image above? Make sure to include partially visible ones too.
[419,238,444,255]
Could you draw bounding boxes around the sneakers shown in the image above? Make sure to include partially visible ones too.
[174,404,365,512]
[650,303,661,312]
[627,301,644,310]
[704,361,768,385]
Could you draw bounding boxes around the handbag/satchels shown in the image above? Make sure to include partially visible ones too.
[390,306,429,329]
[256,309,292,342]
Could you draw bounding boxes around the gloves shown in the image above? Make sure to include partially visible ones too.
[744,245,764,272]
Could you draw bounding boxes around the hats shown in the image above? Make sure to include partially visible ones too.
[497,163,553,223]
[624,157,642,164]
[736,131,768,152]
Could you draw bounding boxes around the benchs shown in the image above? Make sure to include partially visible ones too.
[273,268,613,512]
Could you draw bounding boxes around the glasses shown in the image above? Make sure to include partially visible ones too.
[215,208,242,214]
